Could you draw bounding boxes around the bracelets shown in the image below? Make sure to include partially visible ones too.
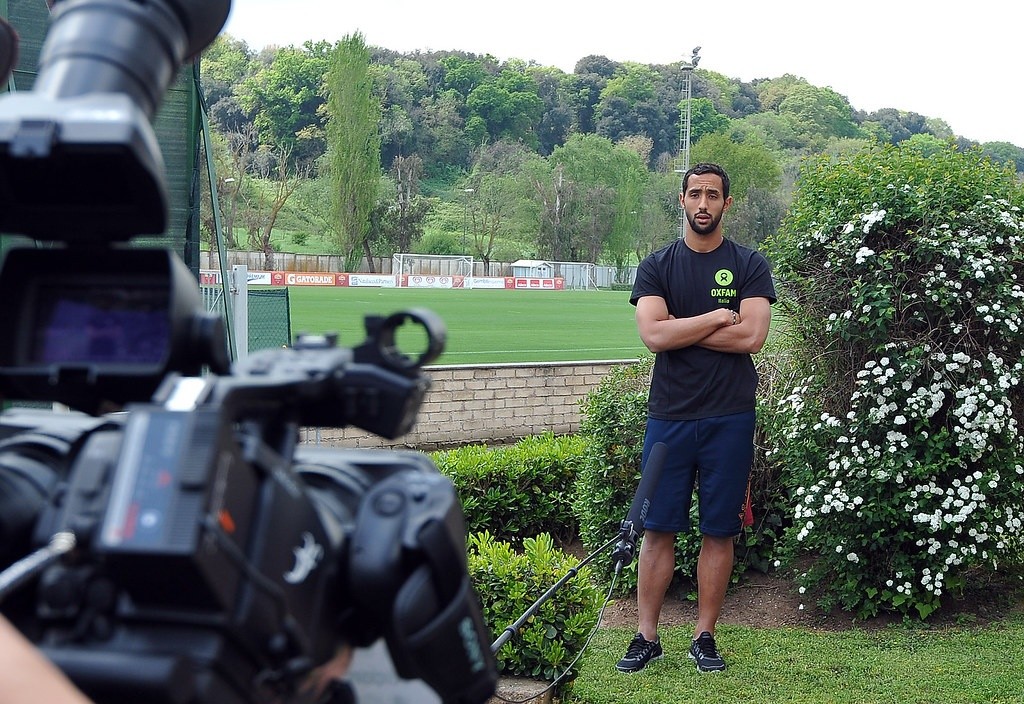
[731,309,735,325]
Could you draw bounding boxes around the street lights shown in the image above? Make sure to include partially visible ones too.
[462,188,475,256]
[225,178,235,268]
[681,45,701,239]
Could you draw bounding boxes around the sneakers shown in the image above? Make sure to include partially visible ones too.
[614,632,664,672]
[688,631,726,676]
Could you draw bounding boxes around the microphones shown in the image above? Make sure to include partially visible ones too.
[611,442,669,576]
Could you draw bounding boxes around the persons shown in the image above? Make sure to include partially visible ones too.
[614,163,777,673]
